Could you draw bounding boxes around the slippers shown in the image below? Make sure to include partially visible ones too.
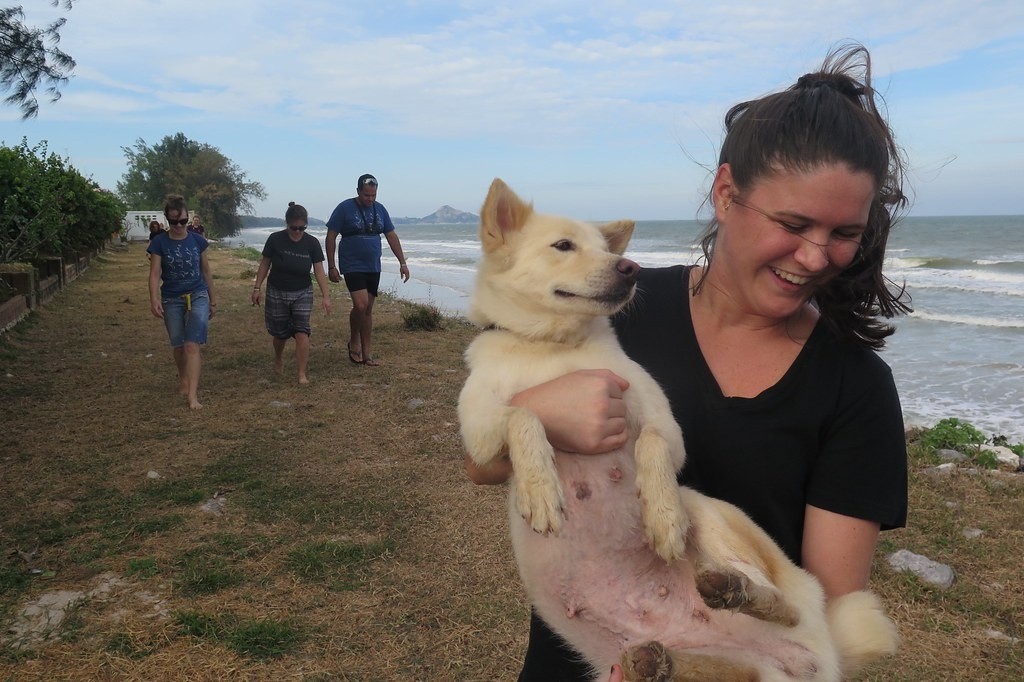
[347,342,362,363]
[364,355,379,366]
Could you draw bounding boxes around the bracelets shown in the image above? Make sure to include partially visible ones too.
[254,287,260,292]
[210,304,216,307]
[401,263,407,267]
[328,266,336,272]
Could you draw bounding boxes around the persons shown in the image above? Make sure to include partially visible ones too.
[251,202,331,384]
[149,221,167,245]
[187,215,205,238]
[465,46,907,682]
[146,195,217,410]
[325,174,410,366]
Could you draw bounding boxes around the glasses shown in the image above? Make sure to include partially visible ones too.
[358,177,378,191]
[287,221,308,232]
[165,214,189,225]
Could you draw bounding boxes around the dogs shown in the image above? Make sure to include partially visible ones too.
[456,177,903,682]
